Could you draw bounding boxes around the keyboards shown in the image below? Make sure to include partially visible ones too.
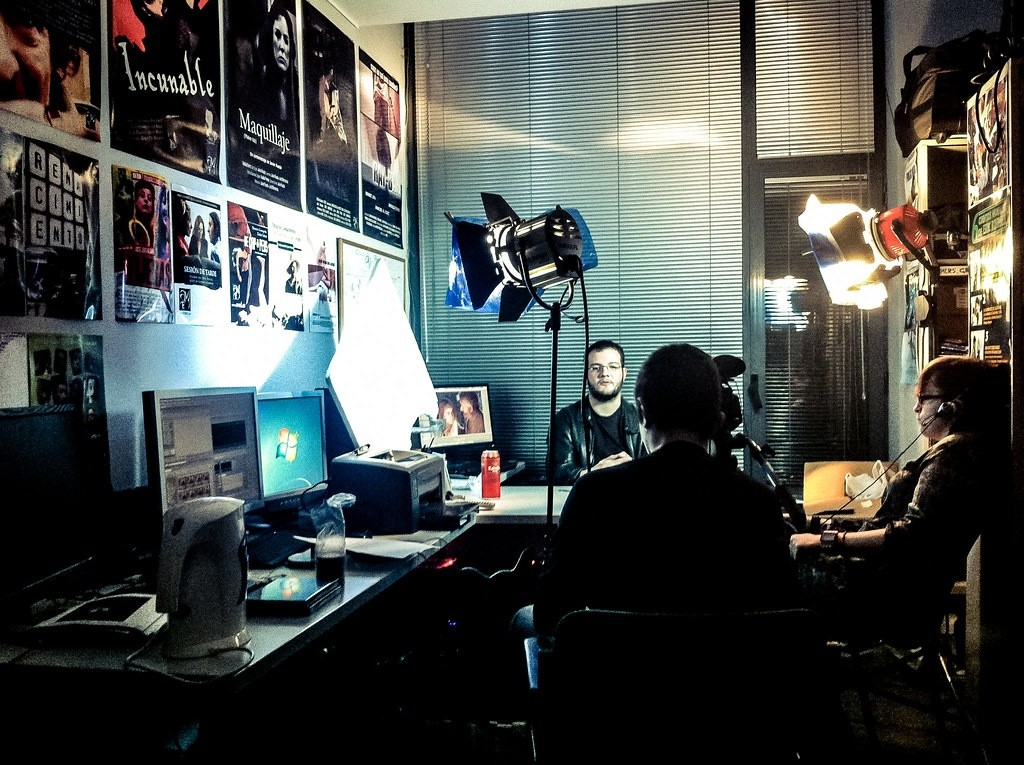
[447,457,517,476]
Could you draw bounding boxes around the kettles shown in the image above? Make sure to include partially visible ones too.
[125,496,254,685]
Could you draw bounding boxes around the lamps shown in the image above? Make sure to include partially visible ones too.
[442,190,599,322]
[796,195,942,311]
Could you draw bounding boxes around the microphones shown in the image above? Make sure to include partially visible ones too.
[920,404,950,426]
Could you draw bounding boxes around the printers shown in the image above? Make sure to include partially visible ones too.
[332,443,447,532]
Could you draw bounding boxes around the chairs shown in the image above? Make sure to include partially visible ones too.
[819,533,991,765]
[519,607,822,765]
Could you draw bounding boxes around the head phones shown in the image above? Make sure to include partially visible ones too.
[936,361,989,418]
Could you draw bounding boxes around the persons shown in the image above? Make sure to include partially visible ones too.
[243,0,300,154]
[436,397,458,438]
[112,173,221,290]
[544,340,648,483]
[283,257,302,297]
[782,359,1003,690]
[0,0,87,136]
[456,392,484,435]
[31,347,102,405]
[507,342,800,697]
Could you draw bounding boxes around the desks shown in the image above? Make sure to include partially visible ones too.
[1,512,478,683]
[445,484,579,525]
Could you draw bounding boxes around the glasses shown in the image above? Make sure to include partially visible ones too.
[917,394,945,404]
[587,362,622,372]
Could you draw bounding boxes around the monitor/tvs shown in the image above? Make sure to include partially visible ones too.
[416,382,496,453]
[0,401,97,601]
[257,389,328,500]
[142,385,265,528]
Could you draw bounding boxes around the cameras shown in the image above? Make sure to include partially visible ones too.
[712,353,747,447]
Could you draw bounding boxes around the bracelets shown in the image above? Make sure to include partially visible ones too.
[819,530,840,556]
[835,531,848,556]
